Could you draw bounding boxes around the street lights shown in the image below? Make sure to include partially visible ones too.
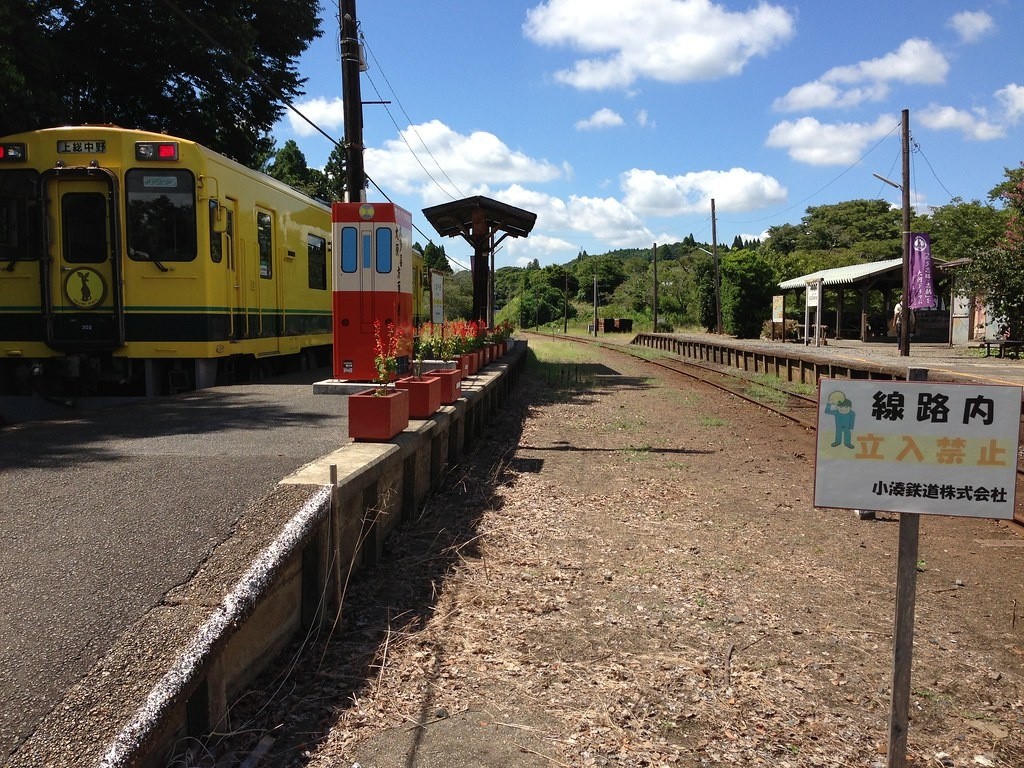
[872,172,910,356]
[699,247,722,336]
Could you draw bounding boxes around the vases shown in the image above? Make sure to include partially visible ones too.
[347,344,519,443]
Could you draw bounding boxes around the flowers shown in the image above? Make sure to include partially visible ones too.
[371,315,523,394]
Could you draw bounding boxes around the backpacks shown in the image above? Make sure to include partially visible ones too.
[898,302,912,322]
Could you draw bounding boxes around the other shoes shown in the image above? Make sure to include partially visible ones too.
[898,348,901,351]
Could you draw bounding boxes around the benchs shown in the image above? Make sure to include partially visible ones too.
[985,339,1021,358]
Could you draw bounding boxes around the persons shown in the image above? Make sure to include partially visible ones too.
[893,294,915,352]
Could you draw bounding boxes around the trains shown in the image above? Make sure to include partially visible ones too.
[0,123,424,400]
